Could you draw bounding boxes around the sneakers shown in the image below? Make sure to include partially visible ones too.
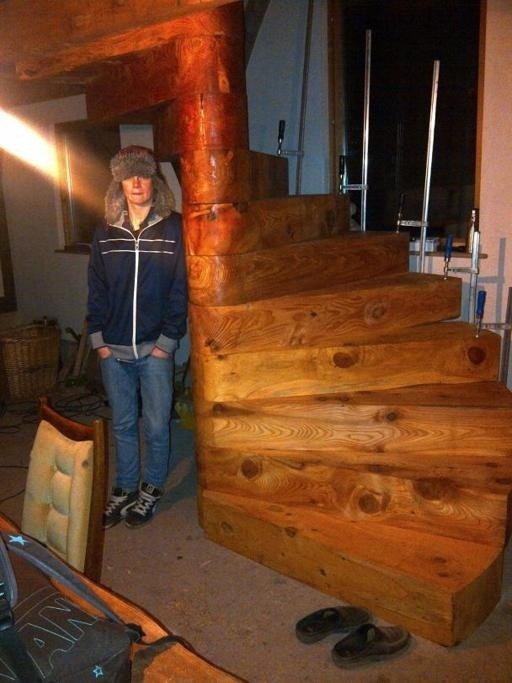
[295,605,370,645]
[331,623,411,670]
[103,486,139,529]
[124,482,162,529]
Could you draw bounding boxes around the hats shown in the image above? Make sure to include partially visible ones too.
[104,145,174,225]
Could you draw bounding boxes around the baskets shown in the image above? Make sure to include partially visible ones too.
[0,325,61,397]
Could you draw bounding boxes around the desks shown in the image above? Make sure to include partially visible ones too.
[0,510,249,683]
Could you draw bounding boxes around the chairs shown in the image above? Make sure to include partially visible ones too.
[21,396,109,585]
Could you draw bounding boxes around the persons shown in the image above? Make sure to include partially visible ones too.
[85,145,188,530]
